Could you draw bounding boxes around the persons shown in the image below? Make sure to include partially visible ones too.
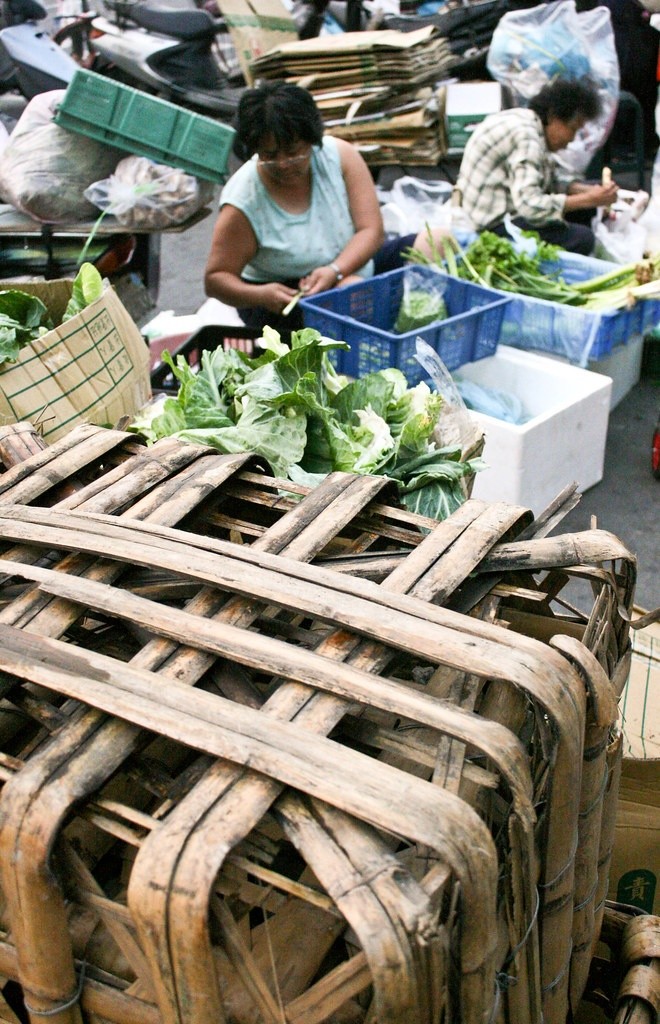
[205,80,384,359]
[457,79,619,256]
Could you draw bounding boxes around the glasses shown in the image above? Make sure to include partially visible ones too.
[257,144,308,168]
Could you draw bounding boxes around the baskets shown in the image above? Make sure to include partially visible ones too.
[438,242,660,361]
[298,264,514,387]
[51,69,238,186]
[0,420,660,1024]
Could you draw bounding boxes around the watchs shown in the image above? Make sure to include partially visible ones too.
[329,263,344,286]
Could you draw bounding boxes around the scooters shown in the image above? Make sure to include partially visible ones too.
[288,0,524,81]
[84,0,347,127]
[0,0,85,112]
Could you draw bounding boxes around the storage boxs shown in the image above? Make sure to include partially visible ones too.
[414,341,613,516]
[55,68,237,188]
[443,232,660,403]
[297,261,510,391]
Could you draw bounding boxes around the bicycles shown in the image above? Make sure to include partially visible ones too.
[51,0,231,74]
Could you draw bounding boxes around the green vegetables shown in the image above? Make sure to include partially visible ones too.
[0,260,470,536]
[399,222,659,305]
